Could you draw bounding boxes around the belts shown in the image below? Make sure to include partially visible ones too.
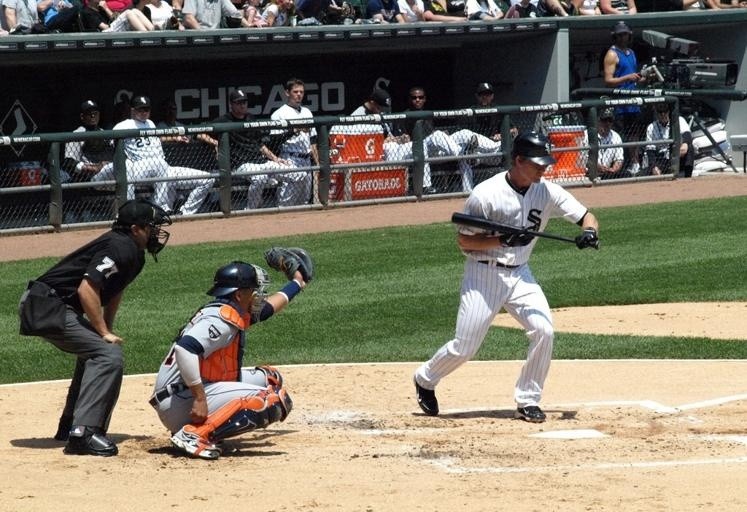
[149,384,179,407]
[28,280,36,288]
[289,150,311,160]
[478,260,520,268]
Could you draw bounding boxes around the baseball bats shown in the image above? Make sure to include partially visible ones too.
[451,212,600,249]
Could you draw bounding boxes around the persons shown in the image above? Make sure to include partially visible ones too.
[646,101,695,178]
[602,22,643,178]
[396,88,479,195]
[0,1,746,36]
[149,254,306,459]
[17,197,173,457]
[350,89,437,197]
[198,80,319,213]
[65,93,216,218]
[597,108,624,176]
[455,81,518,167]
[414,127,601,423]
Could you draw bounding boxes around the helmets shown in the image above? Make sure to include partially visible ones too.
[609,21,633,49]
[206,260,272,324]
[512,130,557,166]
[115,199,172,262]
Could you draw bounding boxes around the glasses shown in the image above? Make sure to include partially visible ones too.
[234,101,246,105]
[601,119,614,123]
[658,109,669,113]
[478,92,493,96]
[410,95,424,100]
[136,109,150,113]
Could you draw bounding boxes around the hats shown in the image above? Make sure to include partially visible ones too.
[130,97,152,109]
[474,83,494,92]
[230,90,248,102]
[371,89,393,112]
[79,101,100,113]
[598,109,616,119]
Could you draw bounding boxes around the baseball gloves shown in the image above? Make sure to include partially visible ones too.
[266,247,311,283]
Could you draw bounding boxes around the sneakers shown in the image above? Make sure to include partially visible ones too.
[56,421,71,441]
[415,375,439,416]
[514,406,546,422]
[424,184,449,194]
[64,426,118,457]
[152,25,163,42]
[464,134,478,169]
[165,16,179,30]
[171,424,224,459]
[167,209,183,215]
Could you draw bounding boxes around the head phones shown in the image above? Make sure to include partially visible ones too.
[609,23,633,43]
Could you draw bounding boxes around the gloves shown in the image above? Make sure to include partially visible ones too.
[498,226,535,248]
[580,227,597,246]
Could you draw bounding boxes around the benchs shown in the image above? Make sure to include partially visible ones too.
[59,125,517,224]
